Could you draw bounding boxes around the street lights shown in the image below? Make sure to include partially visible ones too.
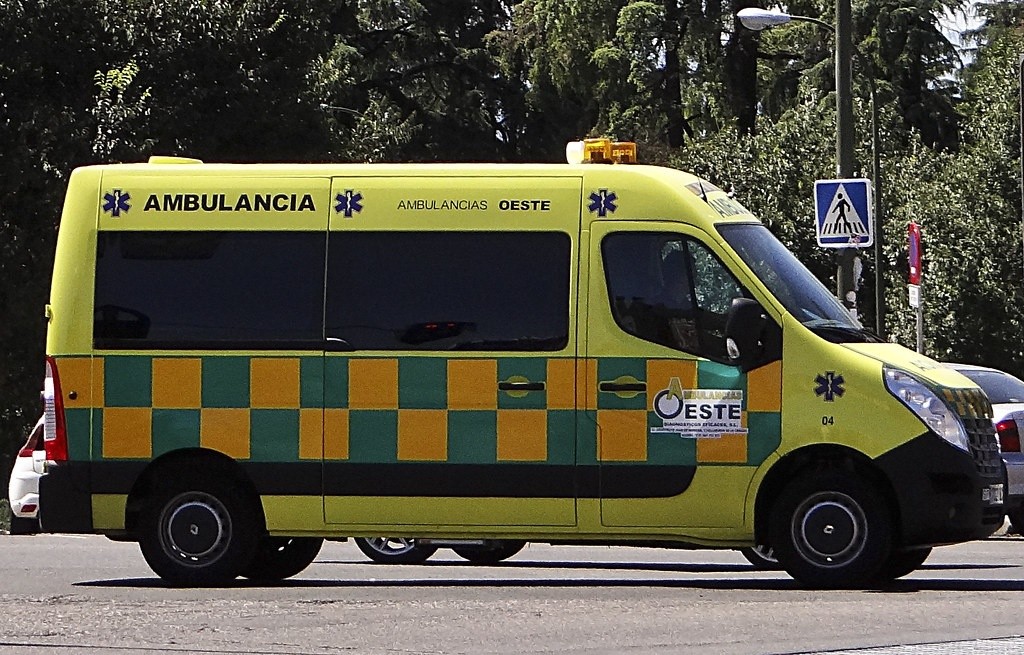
[736,6,888,340]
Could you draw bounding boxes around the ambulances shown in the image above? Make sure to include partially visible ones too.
[39,141,1011,594]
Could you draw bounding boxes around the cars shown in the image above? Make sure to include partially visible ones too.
[7,412,47,533]
[939,360,1024,515]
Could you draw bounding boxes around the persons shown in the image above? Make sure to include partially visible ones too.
[650,251,730,364]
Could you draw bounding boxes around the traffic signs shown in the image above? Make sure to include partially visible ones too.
[813,179,873,248]
